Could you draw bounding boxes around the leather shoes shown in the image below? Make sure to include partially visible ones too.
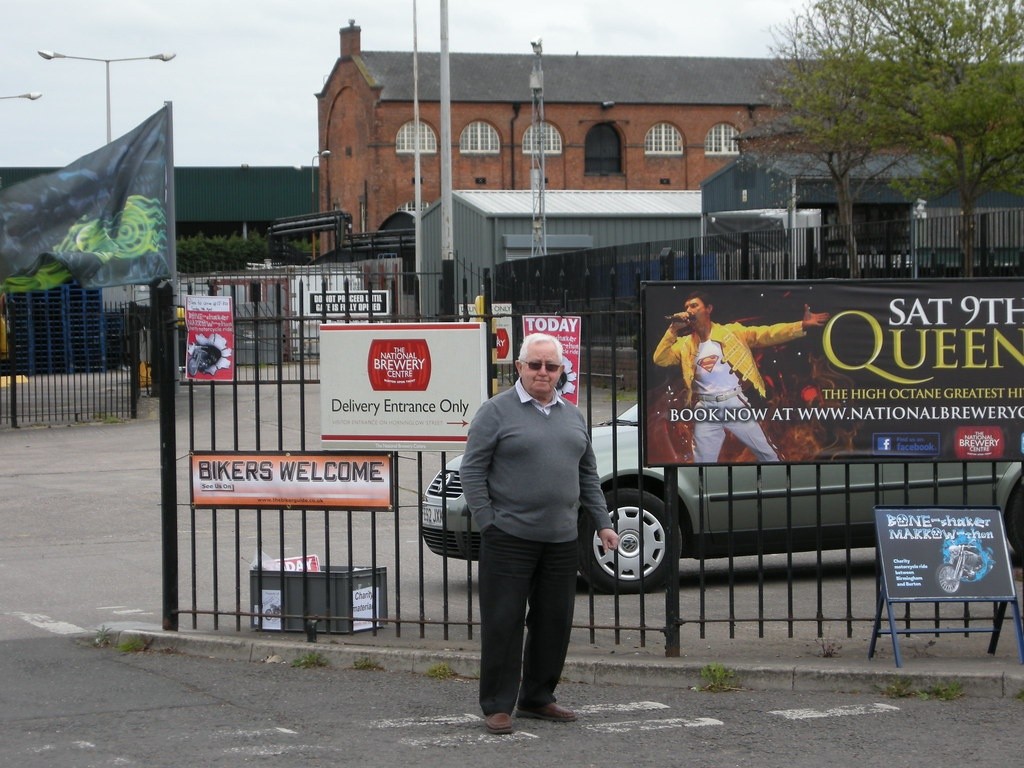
[485,713,514,734]
[515,702,576,722]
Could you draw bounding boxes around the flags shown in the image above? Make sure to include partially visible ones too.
[0,107,167,294]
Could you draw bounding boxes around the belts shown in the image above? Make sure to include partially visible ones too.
[697,387,743,402]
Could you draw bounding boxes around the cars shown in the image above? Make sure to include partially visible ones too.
[422,400,1024,593]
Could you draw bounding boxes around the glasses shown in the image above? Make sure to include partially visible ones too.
[520,359,562,372]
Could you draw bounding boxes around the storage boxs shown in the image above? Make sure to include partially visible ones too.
[250,566,388,633]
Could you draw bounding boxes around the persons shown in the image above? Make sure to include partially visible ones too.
[459,333,620,733]
[653,292,829,462]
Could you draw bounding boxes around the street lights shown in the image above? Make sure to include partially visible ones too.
[311,150,331,259]
[37,50,177,145]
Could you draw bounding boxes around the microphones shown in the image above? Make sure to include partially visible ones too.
[665,313,695,322]
